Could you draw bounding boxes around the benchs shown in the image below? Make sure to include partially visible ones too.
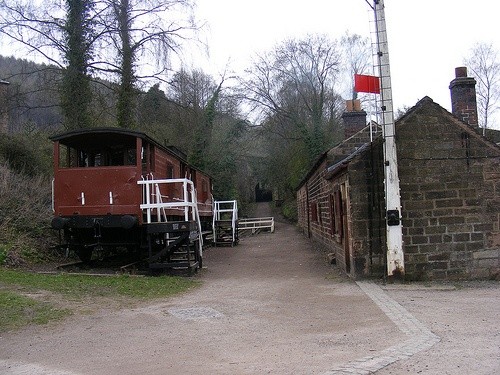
[236,216,275,233]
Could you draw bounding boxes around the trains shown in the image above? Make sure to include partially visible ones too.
[50,127,215,262]
